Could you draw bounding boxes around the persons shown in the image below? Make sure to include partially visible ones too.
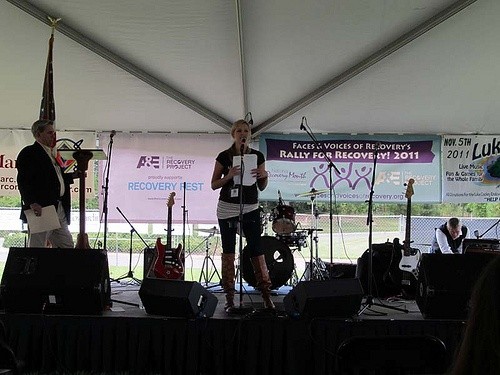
[431,217,471,255]
[16,119,87,249]
[210,120,275,314]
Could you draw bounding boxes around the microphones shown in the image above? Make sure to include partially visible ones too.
[474,230,479,239]
[374,142,381,149]
[300,116,304,130]
[240,136,247,149]
[110,130,116,137]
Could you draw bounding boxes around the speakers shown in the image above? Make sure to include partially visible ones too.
[0,247,111,316]
[283,277,364,320]
[139,278,218,319]
[414,253,497,320]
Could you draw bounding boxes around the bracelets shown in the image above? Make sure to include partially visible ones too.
[223,177,228,182]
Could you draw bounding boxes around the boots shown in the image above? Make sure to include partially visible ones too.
[221,253,235,313]
[250,255,276,310]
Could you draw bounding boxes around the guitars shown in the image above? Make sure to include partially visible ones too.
[388,178,428,300]
[146,191,185,280]
[72,146,94,248]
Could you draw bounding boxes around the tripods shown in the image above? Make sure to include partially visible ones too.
[110,207,157,284]
[358,149,408,316]
[298,195,330,283]
[184,233,221,287]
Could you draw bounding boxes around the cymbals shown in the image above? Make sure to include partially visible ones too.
[193,228,220,233]
[307,212,326,215]
[300,188,327,197]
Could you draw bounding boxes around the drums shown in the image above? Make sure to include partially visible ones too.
[277,229,308,246]
[239,235,294,290]
[272,205,297,233]
[236,204,268,234]
